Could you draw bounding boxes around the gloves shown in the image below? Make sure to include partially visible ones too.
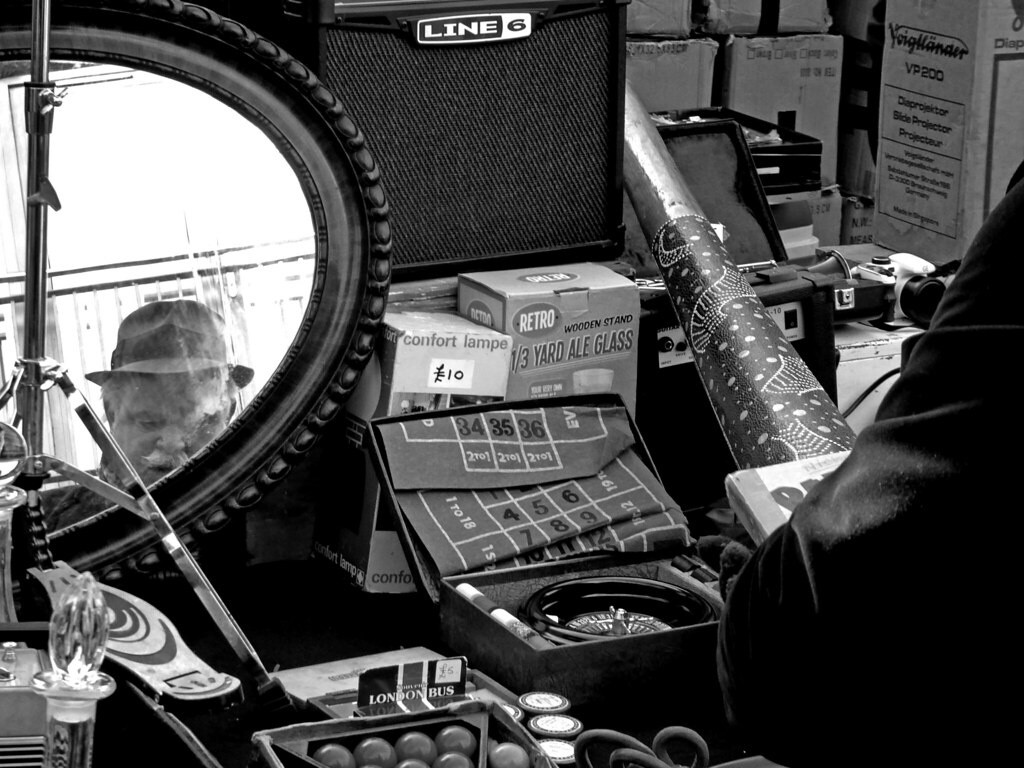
[720,538,751,608]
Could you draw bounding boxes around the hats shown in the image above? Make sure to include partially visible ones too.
[85,298,255,391]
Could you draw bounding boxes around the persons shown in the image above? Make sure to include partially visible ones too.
[40,299,254,533]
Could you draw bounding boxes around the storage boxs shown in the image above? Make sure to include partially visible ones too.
[314,305,514,594]
[458,262,640,421]
[627,0,1024,265]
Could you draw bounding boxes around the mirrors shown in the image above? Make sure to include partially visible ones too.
[0,0,392,615]
